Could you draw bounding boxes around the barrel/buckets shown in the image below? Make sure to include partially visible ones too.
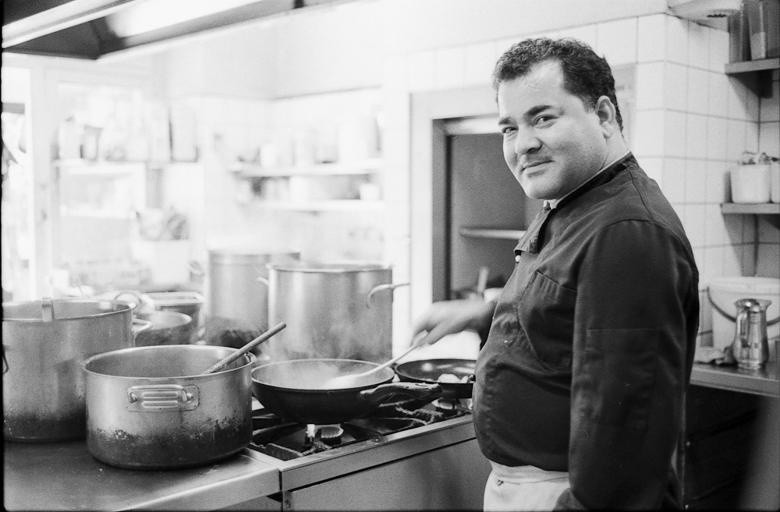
[707,274,780,354]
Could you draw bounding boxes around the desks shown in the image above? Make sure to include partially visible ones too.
[677,355,780,512]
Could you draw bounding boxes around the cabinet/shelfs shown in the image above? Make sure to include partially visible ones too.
[719,57,780,218]
[29,68,414,356]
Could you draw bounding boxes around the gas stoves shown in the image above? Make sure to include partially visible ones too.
[245,402,475,460]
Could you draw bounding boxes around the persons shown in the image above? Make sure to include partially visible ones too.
[407,34,703,512]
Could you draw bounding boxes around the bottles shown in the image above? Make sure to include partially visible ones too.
[731,297,772,372]
[726,1,769,63]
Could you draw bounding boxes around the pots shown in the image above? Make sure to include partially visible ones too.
[250,359,442,428]
[394,356,477,400]
[200,246,300,340]
[79,343,252,474]
[256,263,409,363]
[3,293,141,444]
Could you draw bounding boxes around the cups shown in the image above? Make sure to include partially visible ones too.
[730,164,771,205]
[770,162,780,203]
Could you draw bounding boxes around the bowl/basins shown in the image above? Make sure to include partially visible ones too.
[134,310,193,345]
